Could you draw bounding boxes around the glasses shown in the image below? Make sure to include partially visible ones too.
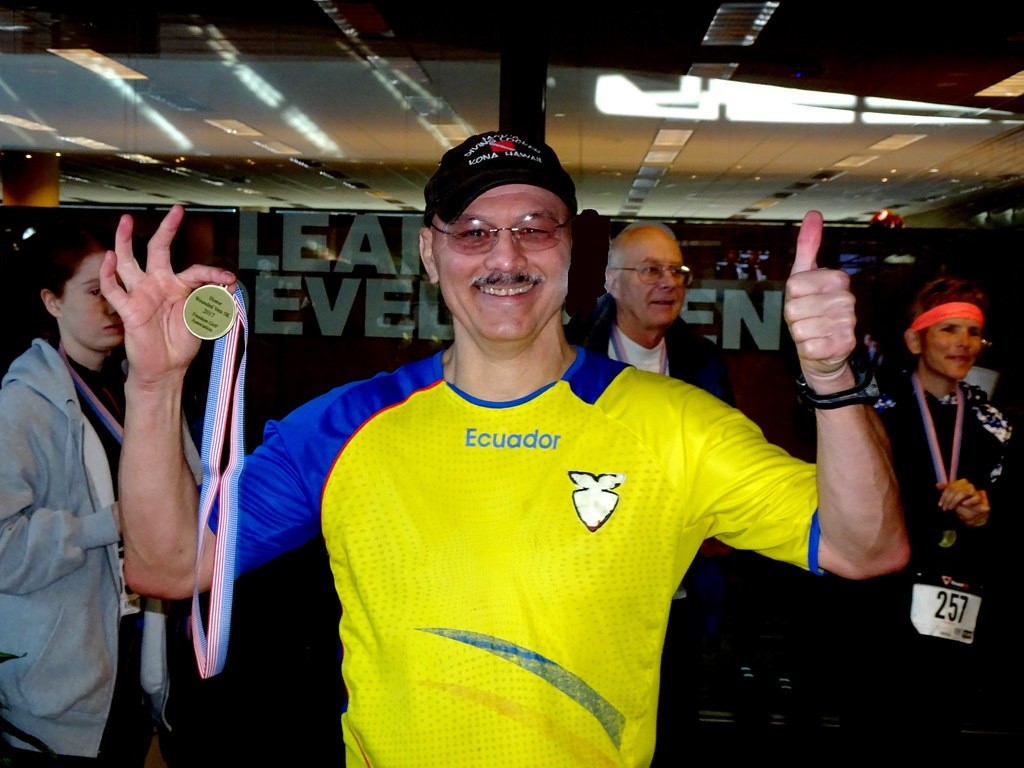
[614,262,694,287]
[430,213,570,256]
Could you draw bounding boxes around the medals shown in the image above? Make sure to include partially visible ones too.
[938,530,957,547]
[183,281,240,341]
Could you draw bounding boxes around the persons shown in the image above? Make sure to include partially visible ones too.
[99,130,911,768]
[0,232,201,768]
[563,224,737,768]
[878,277,1024,768]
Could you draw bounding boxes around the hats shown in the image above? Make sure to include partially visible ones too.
[424,131,578,225]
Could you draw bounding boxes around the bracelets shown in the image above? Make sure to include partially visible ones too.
[798,370,882,409]
